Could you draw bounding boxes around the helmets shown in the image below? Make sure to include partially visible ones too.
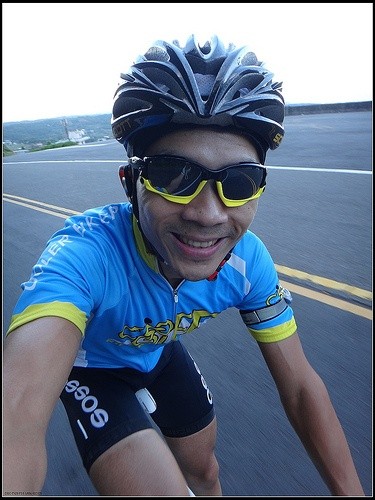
[111,34,285,163]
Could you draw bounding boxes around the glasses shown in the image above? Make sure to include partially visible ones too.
[127,153,267,208]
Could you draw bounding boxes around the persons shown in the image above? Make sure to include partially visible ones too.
[4,32,364,498]
